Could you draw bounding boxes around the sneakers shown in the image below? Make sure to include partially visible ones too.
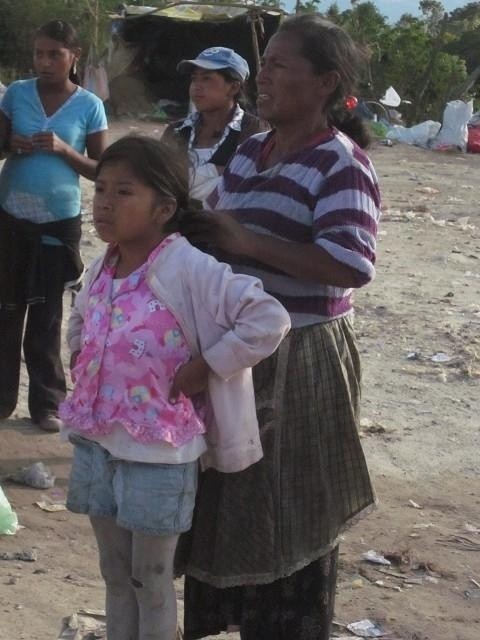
[39,414,62,431]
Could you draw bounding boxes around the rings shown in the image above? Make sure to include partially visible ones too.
[18,147,21,155]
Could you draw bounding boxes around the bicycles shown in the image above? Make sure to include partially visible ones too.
[360,96,391,123]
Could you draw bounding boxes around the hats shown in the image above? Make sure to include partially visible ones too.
[176,46,251,84]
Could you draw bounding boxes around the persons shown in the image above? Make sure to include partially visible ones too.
[0,19,112,430]
[173,15,382,640]
[164,45,266,203]
[59,130,291,640]
